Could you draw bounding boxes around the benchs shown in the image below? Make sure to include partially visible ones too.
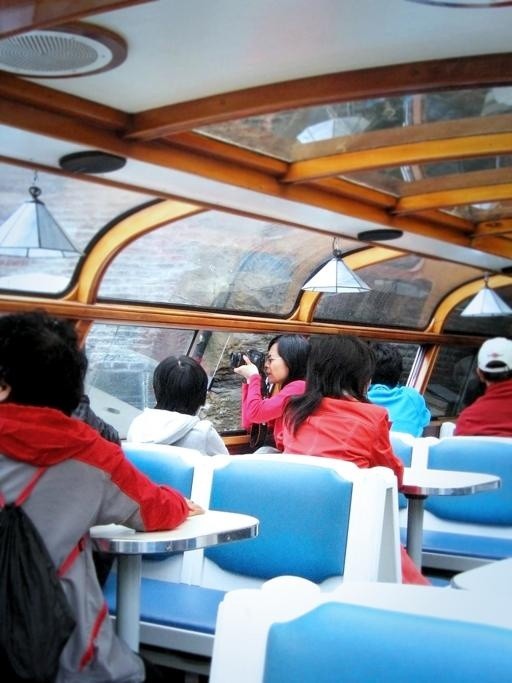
[388,432,512,573]
[207,575,512,683]
[99,445,385,656]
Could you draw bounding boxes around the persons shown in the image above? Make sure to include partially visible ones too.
[453,337,512,439]
[233,332,308,454]
[126,354,230,457]
[0,315,203,683]
[363,340,431,439]
[20,310,121,589]
[282,333,431,586]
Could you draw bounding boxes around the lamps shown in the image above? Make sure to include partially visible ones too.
[300,236,374,294]
[0,168,86,258]
[461,273,512,318]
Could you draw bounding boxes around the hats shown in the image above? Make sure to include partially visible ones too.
[477,337,512,373]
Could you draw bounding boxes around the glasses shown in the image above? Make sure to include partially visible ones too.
[268,357,283,362]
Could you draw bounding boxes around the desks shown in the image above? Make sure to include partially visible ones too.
[86,510,262,648]
[395,467,503,572]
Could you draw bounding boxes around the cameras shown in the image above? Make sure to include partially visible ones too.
[229,348,265,372]
